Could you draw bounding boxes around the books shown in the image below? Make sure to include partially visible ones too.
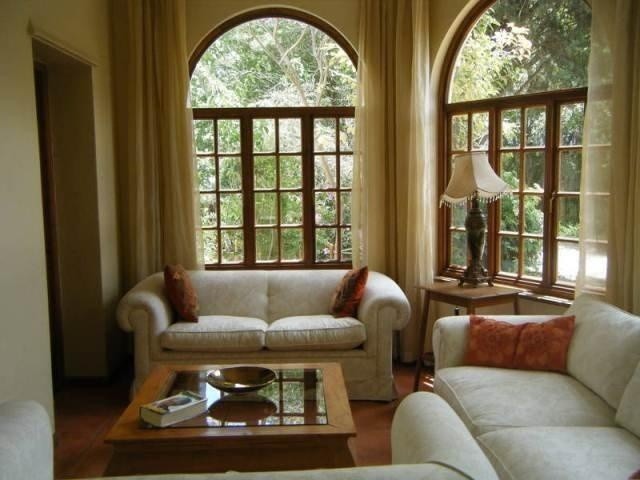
[138,390,209,429]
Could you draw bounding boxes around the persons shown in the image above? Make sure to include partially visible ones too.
[149,397,192,411]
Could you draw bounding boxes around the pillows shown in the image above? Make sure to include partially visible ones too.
[563,293,640,410]
[463,313,576,369]
[613,361,640,438]
[329,264,368,318]
[165,264,201,323]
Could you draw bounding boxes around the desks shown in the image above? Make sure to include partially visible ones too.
[413,281,524,392]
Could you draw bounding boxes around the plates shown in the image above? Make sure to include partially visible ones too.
[208,393,278,421]
[207,366,278,392]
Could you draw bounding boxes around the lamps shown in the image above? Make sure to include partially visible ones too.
[439,151,514,288]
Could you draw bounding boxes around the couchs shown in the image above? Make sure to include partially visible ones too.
[432,315,640,480]
[115,269,412,402]
[86,391,500,480]
